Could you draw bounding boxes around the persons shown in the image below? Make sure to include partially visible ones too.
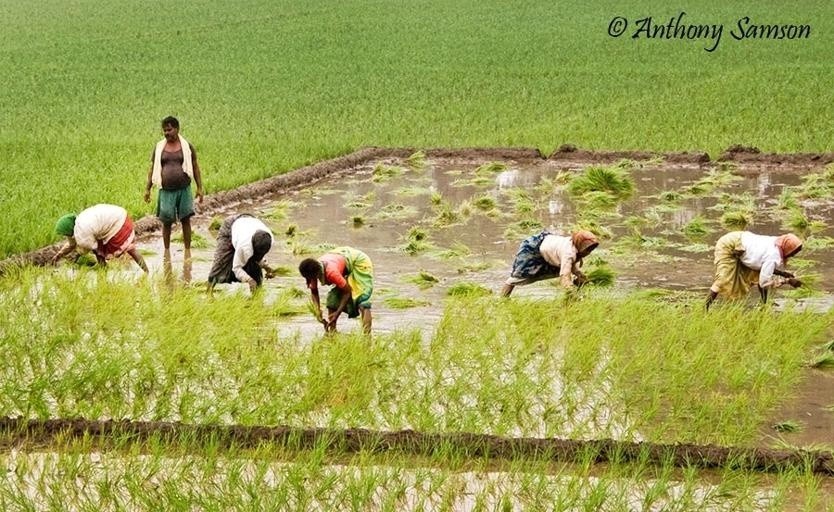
[297,245,373,332]
[208,212,275,286]
[704,231,804,308]
[499,229,599,296]
[50,205,149,275]
[143,115,203,249]
